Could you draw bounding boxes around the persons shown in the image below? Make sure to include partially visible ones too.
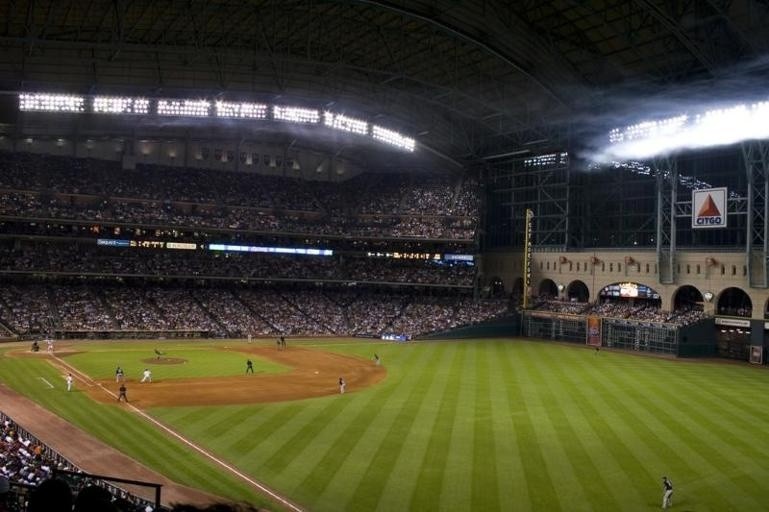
[280,333,287,350]
[247,333,251,343]
[659,475,675,509]
[371,351,381,366]
[116,384,129,403]
[244,359,255,376]
[31,340,38,352]
[595,342,603,354]
[140,367,153,384]
[336,377,345,394]
[2,149,513,341]
[66,372,73,392]
[46,335,54,353]
[0,413,264,510]
[115,365,124,384]
[512,288,752,326]
